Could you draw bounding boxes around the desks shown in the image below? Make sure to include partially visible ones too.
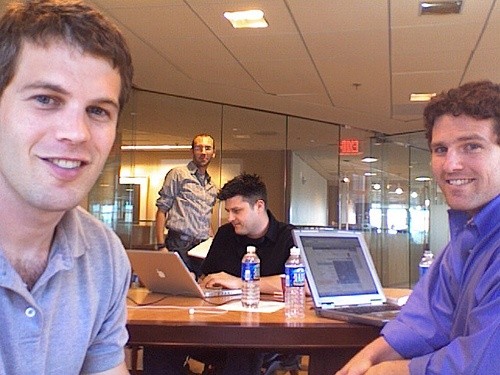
[124,287,414,375]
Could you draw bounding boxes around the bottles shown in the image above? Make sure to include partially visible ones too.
[284,247,305,318]
[418,250,433,276]
[240,246,261,308]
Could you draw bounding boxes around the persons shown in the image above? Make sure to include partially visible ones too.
[155,135,220,281]
[0,0,132,375]
[333,79,500,375]
[143,171,298,375]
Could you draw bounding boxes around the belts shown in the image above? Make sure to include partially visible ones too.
[168,234,205,245]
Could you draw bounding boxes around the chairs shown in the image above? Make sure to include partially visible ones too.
[191,258,300,375]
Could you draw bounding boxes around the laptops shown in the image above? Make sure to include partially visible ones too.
[125,249,243,298]
[291,229,404,326]
